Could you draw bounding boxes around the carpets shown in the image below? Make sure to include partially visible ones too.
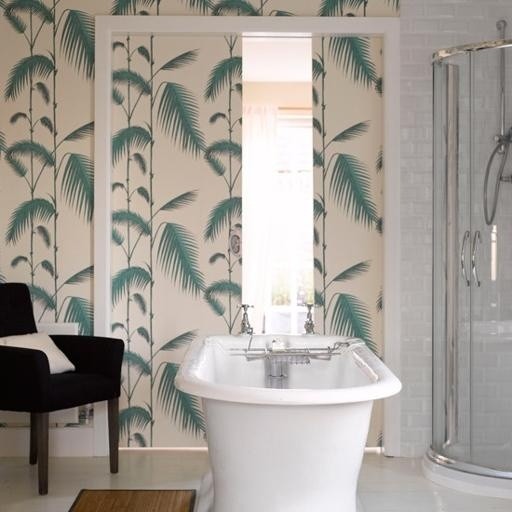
[65,488,198,512]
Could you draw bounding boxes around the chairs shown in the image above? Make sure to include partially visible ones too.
[1,281,127,494]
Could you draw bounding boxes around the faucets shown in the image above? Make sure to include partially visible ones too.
[237,303,255,338]
[303,303,315,334]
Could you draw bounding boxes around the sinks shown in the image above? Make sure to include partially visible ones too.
[173,334,404,512]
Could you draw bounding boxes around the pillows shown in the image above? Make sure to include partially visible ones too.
[1,328,77,377]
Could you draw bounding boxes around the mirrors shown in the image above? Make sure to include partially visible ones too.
[239,31,317,330]
